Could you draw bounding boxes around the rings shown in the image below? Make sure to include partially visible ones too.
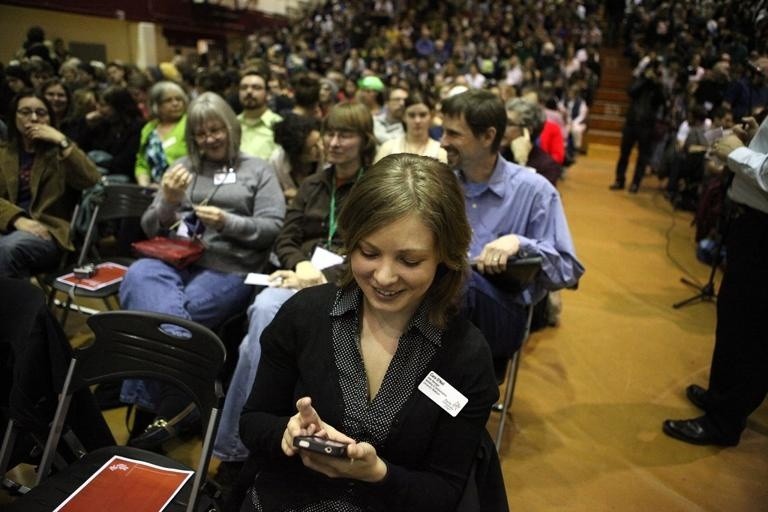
[492,258,499,261]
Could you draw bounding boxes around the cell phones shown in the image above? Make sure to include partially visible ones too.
[293,436,348,458]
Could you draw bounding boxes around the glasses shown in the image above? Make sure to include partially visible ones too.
[191,128,224,141]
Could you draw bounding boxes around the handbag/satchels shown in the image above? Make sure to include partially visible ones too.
[131,236,204,270]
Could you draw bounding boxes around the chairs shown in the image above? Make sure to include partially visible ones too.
[47,185,159,335]
[2,309,228,512]
[0,277,45,475]
[486,304,537,453]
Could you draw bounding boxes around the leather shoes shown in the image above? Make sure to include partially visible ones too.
[687,385,708,411]
[663,413,740,447]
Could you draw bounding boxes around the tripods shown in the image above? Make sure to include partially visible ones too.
[673,235,723,309]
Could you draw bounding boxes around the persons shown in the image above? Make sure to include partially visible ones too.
[113,91,286,453]
[433,87,587,400]
[202,102,378,460]
[2,92,106,276]
[661,118,768,447]
[239,152,510,511]
[1,1,602,202]
[608,2,767,240]
